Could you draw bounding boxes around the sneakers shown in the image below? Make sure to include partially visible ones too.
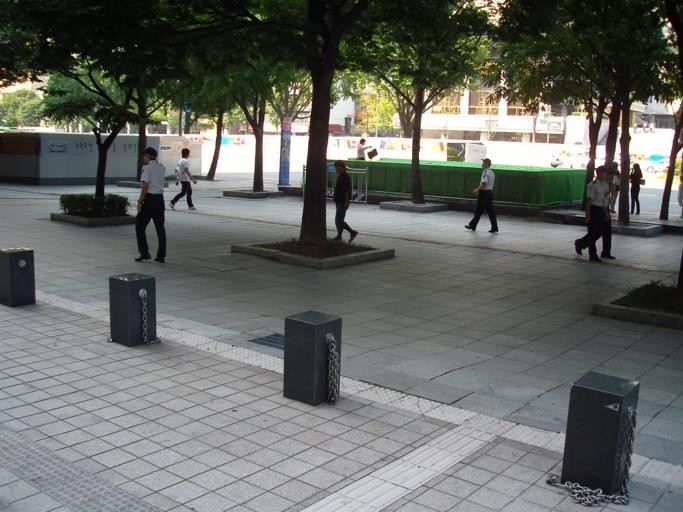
[488,228,499,233]
[348,230,358,242]
[186,205,197,210]
[167,201,175,212]
[135,252,152,262]
[153,256,165,264]
[332,236,343,241]
[574,239,583,256]
[589,255,603,263]
[601,251,615,260]
[464,225,477,231]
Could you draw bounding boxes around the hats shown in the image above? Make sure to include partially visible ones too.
[141,147,157,160]
[481,158,492,167]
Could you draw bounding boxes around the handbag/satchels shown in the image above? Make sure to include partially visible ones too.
[638,177,645,185]
[367,147,378,159]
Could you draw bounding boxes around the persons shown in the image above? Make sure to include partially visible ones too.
[582,168,616,259]
[608,160,621,213]
[134,147,166,263]
[169,147,197,209]
[465,158,499,232]
[333,161,358,242]
[630,164,643,214]
[575,166,608,261]
[357,138,371,159]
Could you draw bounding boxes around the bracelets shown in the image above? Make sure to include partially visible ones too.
[137,199,143,203]
